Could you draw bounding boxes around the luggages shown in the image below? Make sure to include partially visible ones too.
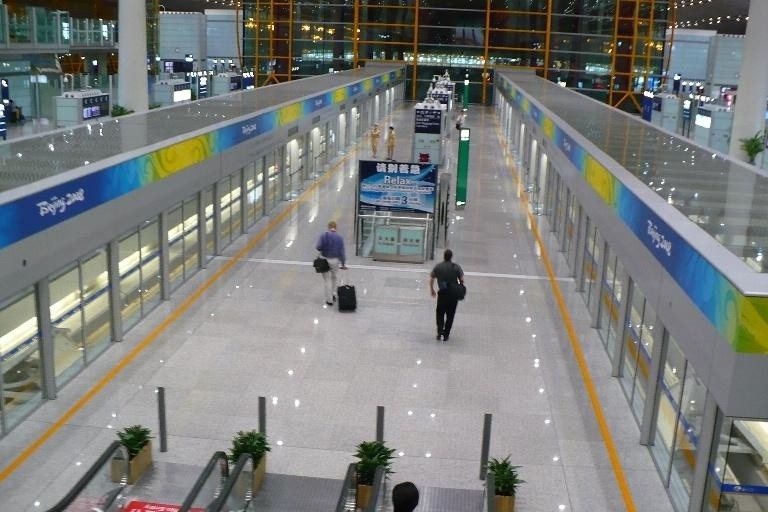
[336,266,356,312]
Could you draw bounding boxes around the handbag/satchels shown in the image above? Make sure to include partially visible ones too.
[312,257,329,273]
[446,282,465,300]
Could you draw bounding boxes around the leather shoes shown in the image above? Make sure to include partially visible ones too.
[437,331,443,340]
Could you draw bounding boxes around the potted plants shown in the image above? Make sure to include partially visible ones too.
[482,454,526,511]
[111,425,153,486]
[354,441,396,510]
[227,430,271,500]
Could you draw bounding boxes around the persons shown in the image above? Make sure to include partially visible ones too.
[429,248,464,341]
[370,122,378,158]
[390,482,419,512]
[316,221,345,305]
[385,126,395,159]
